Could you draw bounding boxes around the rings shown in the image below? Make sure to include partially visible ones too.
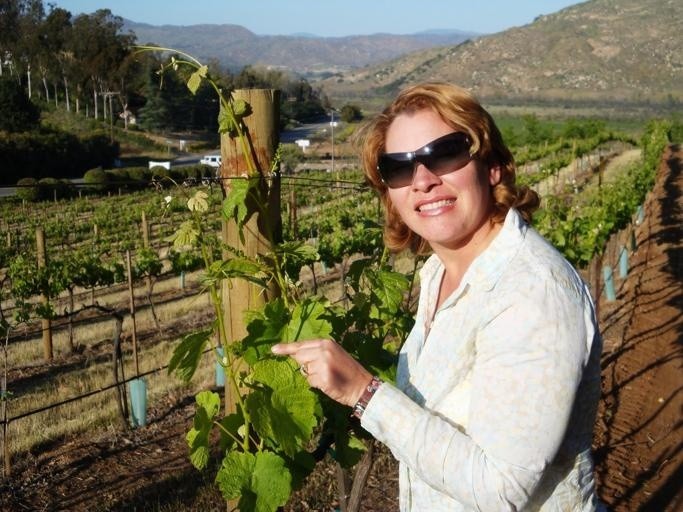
[297,366,311,378]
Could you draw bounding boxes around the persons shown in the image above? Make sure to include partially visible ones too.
[269,79,605,512]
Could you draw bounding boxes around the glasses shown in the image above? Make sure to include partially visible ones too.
[378,131,475,189]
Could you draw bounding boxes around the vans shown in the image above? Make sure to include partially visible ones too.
[199,155,222,168]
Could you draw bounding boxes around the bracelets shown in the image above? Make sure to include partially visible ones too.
[350,371,385,423]
